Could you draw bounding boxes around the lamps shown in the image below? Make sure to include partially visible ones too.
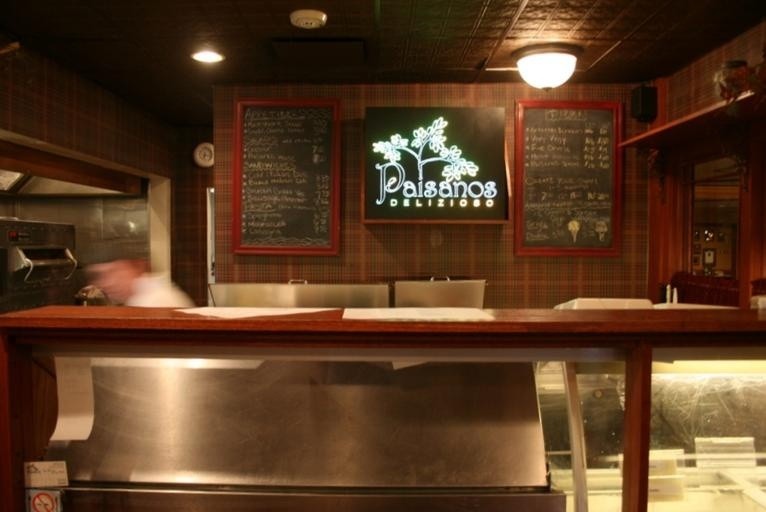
[508,40,584,96]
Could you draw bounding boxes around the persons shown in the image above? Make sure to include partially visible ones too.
[75,248,196,308]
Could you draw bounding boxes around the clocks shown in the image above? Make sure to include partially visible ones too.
[195,139,214,167]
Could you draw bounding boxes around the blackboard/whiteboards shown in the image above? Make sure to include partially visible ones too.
[231,98,342,258]
[512,99,623,259]
